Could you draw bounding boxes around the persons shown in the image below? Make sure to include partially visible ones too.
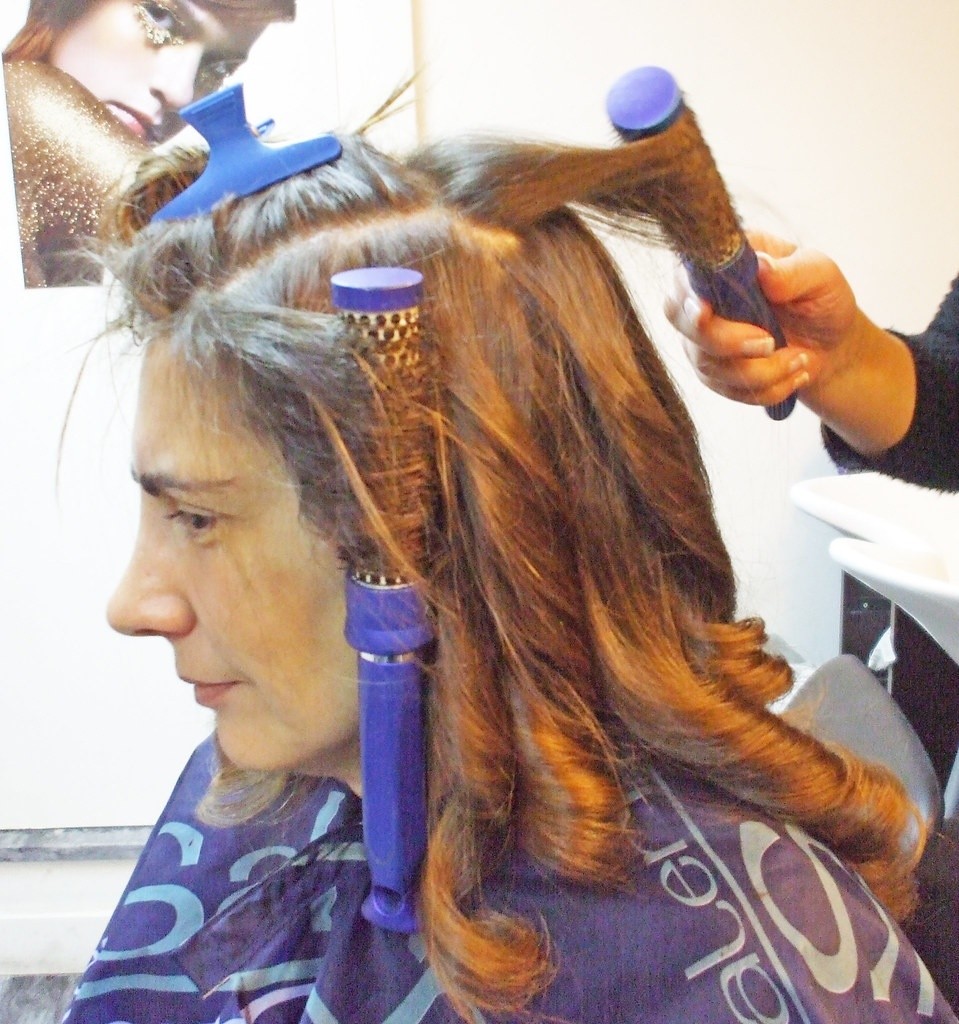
[55,72,959,1024]
[1,0,296,288]
[664,223,959,492]
